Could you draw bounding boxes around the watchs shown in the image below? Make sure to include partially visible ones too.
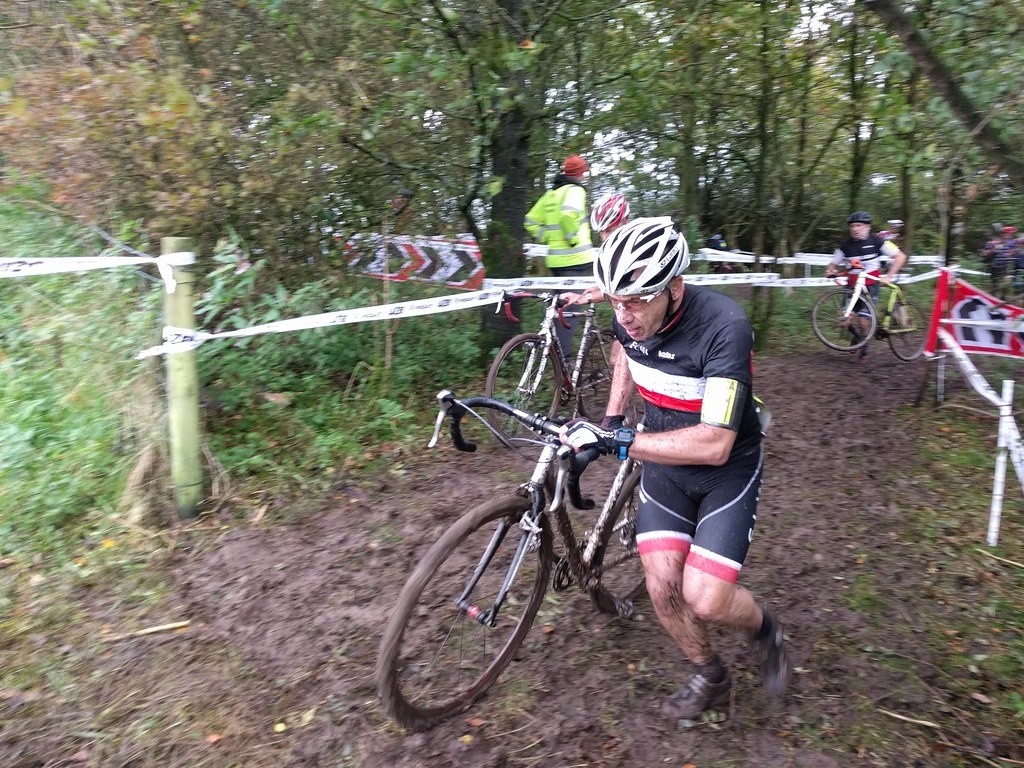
[615,426,635,459]
[585,293,593,305]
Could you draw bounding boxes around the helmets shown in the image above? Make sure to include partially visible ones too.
[590,194,630,231]
[991,223,1003,233]
[846,211,873,222]
[565,156,589,176]
[592,216,690,295]
[1001,227,1018,233]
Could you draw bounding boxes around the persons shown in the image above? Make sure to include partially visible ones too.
[524,156,594,387]
[561,192,797,727]
[978,225,1024,291]
[552,193,640,428]
[827,210,908,360]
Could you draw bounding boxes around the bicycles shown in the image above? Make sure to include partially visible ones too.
[483,289,631,451]
[372,391,663,735]
[808,258,930,362]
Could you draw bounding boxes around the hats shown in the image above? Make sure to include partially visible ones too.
[708,234,722,239]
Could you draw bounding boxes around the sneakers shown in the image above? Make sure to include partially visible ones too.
[663,669,731,722]
[749,602,792,696]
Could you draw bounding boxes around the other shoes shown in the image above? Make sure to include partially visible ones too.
[849,336,869,359]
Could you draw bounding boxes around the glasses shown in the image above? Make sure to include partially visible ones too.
[604,286,667,311]
